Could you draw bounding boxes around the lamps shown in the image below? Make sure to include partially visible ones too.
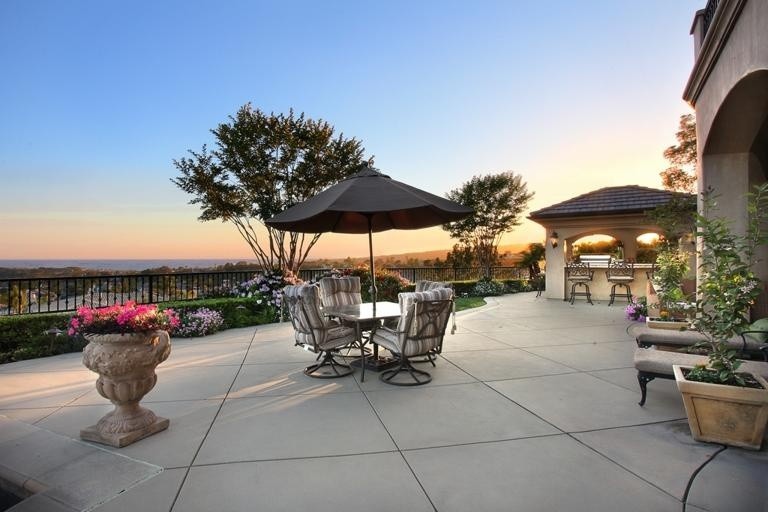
[549,231,559,248]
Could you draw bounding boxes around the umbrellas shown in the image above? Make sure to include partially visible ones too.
[265,161,479,359]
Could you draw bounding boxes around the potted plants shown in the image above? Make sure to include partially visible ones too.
[644,182,766,452]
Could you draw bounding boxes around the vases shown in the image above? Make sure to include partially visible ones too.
[75,328,173,449]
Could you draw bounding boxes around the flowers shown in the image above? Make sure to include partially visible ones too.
[66,302,180,342]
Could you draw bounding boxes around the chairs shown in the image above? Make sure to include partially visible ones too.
[629,327,767,408]
[565,258,635,306]
[282,276,454,387]
